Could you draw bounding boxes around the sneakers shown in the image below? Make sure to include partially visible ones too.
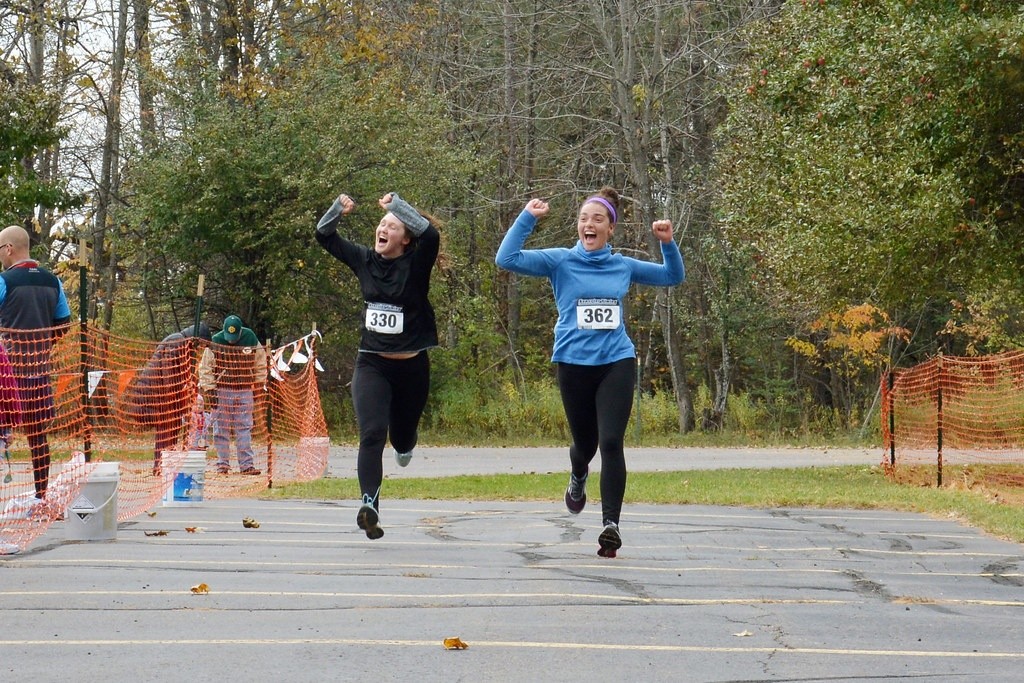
[395,450,412,467]
[356,493,384,539]
[564,473,588,513]
[597,524,622,558]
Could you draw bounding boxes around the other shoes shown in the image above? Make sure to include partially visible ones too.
[219,463,230,469]
[242,466,261,475]
[26,501,48,521]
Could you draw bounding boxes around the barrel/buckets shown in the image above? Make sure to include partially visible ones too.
[298,437,330,475]
[64,463,122,541]
[161,449,206,507]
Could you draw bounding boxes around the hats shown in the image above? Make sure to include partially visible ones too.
[199,322,212,342]
[223,315,242,340]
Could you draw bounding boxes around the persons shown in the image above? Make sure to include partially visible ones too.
[495,186,686,559]
[314,192,451,540]
[127,315,268,477]
[0,225,72,518]
